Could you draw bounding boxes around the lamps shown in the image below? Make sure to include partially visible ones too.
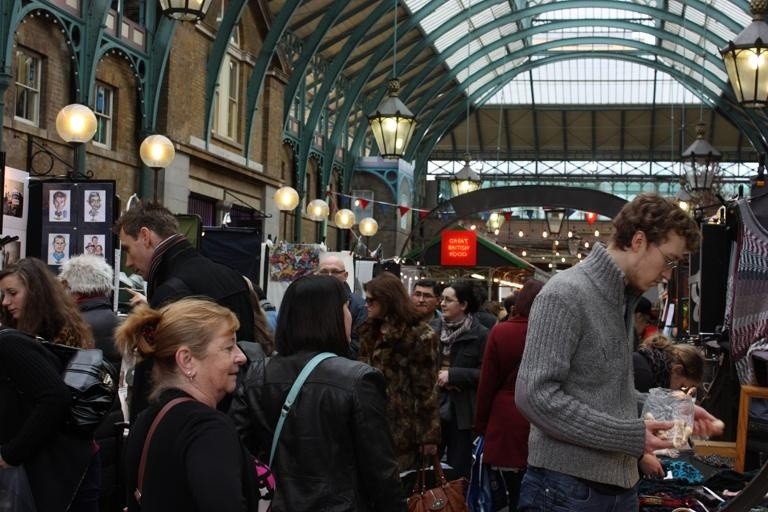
[681,4,722,196]
[158,1,215,23]
[565,234,584,257]
[720,0,768,111]
[486,0,505,234]
[367,0,418,162]
[451,0,483,207]
[543,207,567,236]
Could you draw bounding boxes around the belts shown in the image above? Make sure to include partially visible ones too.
[529,469,647,496]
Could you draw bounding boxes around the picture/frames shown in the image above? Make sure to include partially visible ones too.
[40,178,116,269]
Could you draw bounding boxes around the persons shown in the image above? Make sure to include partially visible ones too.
[428,281,491,476]
[0,319,107,511]
[242,254,709,484]
[515,191,725,511]
[112,295,260,511]
[0,256,95,350]
[270,245,318,281]
[352,271,443,476]
[226,272,409,511]
[48,189,105,262]
[56,252,124,372]
[472,277,546,511]
[113,198,255,345]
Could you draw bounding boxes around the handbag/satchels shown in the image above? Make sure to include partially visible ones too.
[407,458,466,512]
[64,348,119,438]
[466,436,509,512]
[243,449,276,511]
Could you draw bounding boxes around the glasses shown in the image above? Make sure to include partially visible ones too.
[657,246,682,273]
[440,296,459,303]
[415,293,438,298]
[320,270,345,276]
[365,296,376,306]
